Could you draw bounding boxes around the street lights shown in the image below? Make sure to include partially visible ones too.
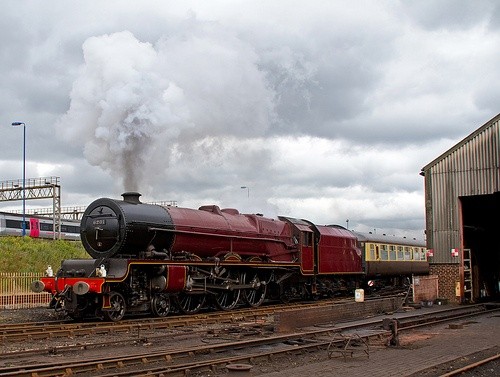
[241,186,250,214]
[12,121,26,238]
[346,218,350,229]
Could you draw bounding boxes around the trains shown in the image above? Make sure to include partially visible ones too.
[0,210,82,241]
[30,192,426,322]
